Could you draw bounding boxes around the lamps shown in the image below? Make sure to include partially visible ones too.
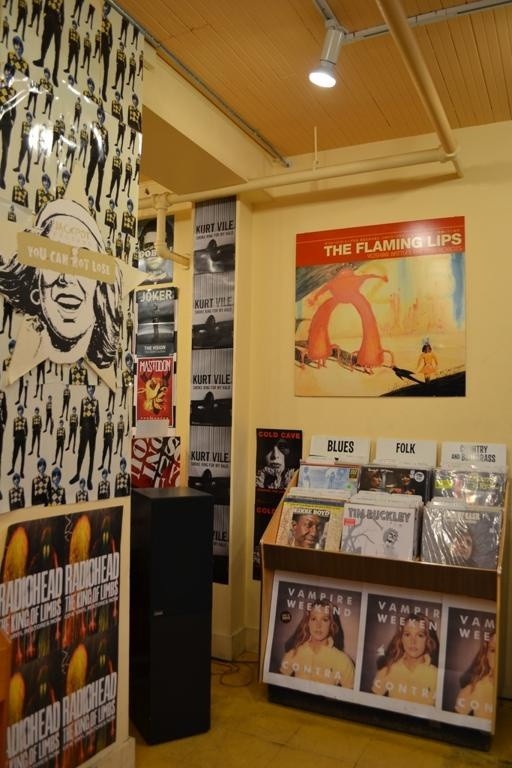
[309,19,348,88]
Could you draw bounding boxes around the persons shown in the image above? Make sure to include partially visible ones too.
[278,597,355,691]
[255,437,296,490]
[138,219,173,286]
[1,1,142,512]
[369,613,438,708]
[417,342,438,384]
[455,631,496,721]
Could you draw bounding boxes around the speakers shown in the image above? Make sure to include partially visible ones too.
[131,487,214,745]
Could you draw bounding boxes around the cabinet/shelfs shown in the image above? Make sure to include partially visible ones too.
[257,468,512,751]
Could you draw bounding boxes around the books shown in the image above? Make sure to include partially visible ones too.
[274,456,508,571]
